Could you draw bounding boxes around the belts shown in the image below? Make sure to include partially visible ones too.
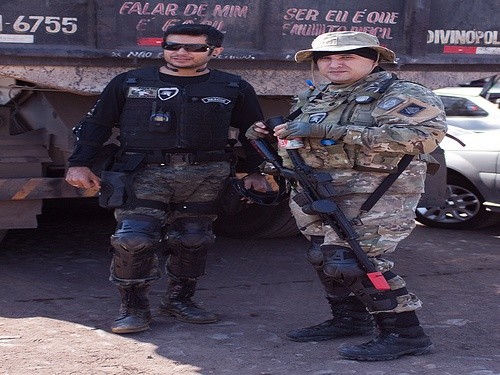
[144,152,226,165]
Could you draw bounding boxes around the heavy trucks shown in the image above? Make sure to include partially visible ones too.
[0,0,500,240]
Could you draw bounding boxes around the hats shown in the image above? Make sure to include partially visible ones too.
[294,30,396,64]
[312,47,381,64]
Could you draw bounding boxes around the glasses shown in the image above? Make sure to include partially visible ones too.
[160,41,222,53]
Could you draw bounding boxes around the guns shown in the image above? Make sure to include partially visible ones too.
[250,116,391,292]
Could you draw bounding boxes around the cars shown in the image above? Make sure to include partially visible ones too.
[413,72,500,230]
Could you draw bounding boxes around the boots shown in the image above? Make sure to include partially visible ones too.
[158,277,218,324]
[336,311,435,361]
[111,286,153,333]
[285,301,376,342]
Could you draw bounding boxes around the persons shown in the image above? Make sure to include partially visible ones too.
[66,24,283,333]
[245,31,448,361]
